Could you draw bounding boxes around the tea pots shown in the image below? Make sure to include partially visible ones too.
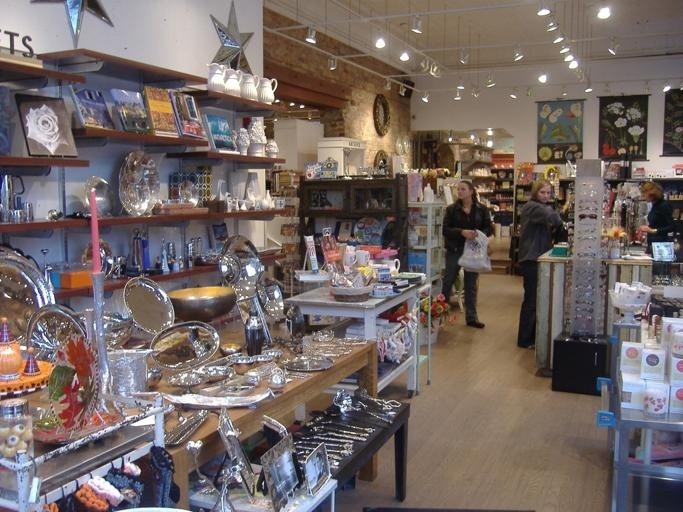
[0,168,25,223]
[380,258,400,273]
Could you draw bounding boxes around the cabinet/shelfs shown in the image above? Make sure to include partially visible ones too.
[587,364,683,511]
[0,44,299,329]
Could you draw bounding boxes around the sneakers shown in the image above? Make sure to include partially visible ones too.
[467,320,484,328]
[518,341,535,349]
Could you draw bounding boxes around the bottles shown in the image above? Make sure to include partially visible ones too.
[424,184,432,203]
[132,228,142,275]
[245,310,263,357]
[266,190,272,208]
[185,237,203,268]
[0,399,34,470]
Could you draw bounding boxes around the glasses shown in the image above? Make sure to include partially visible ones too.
[573,315,592,323]
[578,214,597,222]
[575,269,595,275]
[577,252,596,258]
[575,300,594,306]
[575,291,594,298]
[577,207,598,213]
[575,307,594,314]
[577,228,596,234]
[574,284,595,289]
[577,236,596,242]
[575,277,595,282]
[579,200,599,205]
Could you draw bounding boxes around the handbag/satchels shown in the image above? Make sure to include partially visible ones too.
[554,223,568,244]
[457,229,492,273]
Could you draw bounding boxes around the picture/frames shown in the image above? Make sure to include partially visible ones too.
[373,93,390,137]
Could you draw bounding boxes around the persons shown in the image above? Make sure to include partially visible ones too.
[442,180,494,328]
[517,178,563,349]
[639,181,675,254]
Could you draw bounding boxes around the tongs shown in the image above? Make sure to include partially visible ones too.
[165,408,209,447]
[353,386,402,411]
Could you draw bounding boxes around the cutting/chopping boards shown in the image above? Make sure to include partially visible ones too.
[155,207,210,214]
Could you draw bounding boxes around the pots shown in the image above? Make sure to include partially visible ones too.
[168,286,236,318]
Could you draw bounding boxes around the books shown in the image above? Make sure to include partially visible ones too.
[69,85,116,131]
[203,112,237,151]
[208,222,229,252]
[169,90,208,140]
[14,93,79,157]
[143,85,179,138]
[110,88,150,133]
[345,317,405,338]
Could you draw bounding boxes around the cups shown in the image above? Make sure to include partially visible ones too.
[173,263,180,272]
[261,199,268,211]
[142,232,150,276]
[23,202,33,221]
[345,246,370,265]
[113,257,127,279]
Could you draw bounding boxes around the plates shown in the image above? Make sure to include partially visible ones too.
[332,337,368,345]
[160,393,275,409]
[82,238,114,282]
[149,321,221,371]
[26,304,101,436]
[282,355,334,373]
[124,276,175,336]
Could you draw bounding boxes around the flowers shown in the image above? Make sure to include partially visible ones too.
[25,103,69,155]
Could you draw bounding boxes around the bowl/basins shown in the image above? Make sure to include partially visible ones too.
[196,350,281,382]
[220,343,241,355]
[239,199,254,211]
[85,176,113,216]
[218,235,264,288]
[119,150,160,216]
[158,197,200,209]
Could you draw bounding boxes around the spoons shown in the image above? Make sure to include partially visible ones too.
[294,415,374,443]
